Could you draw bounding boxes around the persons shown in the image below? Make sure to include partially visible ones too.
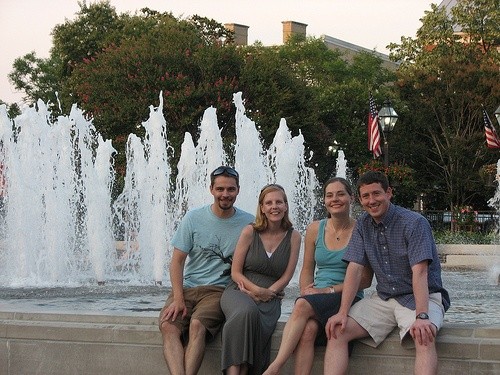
[158,165,256,375]
[323,171,450,375]
[220,184,302,375]
[262,177,373,375]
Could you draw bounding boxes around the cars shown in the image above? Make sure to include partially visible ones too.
[425,191,500,236]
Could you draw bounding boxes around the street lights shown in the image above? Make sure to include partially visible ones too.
[377,107,399,170]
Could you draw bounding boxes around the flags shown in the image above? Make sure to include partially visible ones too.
[482,106,500,149]
[368,93,385,159]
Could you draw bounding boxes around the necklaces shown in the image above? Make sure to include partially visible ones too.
[330,219,351,240]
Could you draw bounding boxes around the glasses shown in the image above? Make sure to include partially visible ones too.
[260,184,285,193]
[212,168,238,176]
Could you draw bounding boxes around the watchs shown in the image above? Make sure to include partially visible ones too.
[416,312,429,320]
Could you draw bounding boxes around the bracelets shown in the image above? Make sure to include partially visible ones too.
[329,286,336,293]
